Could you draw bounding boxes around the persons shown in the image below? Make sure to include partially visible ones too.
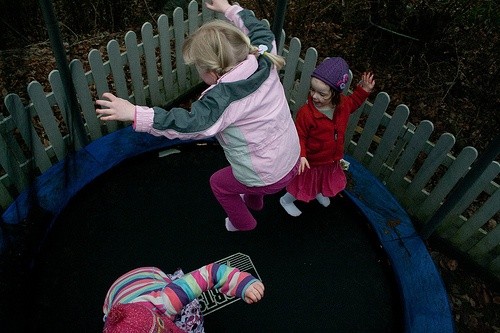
[102,263,265,333]
[279,57,375,217]
[96,0,300,232]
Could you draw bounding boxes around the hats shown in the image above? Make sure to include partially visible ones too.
[310,57,349,92]
[103,301,184,333]
